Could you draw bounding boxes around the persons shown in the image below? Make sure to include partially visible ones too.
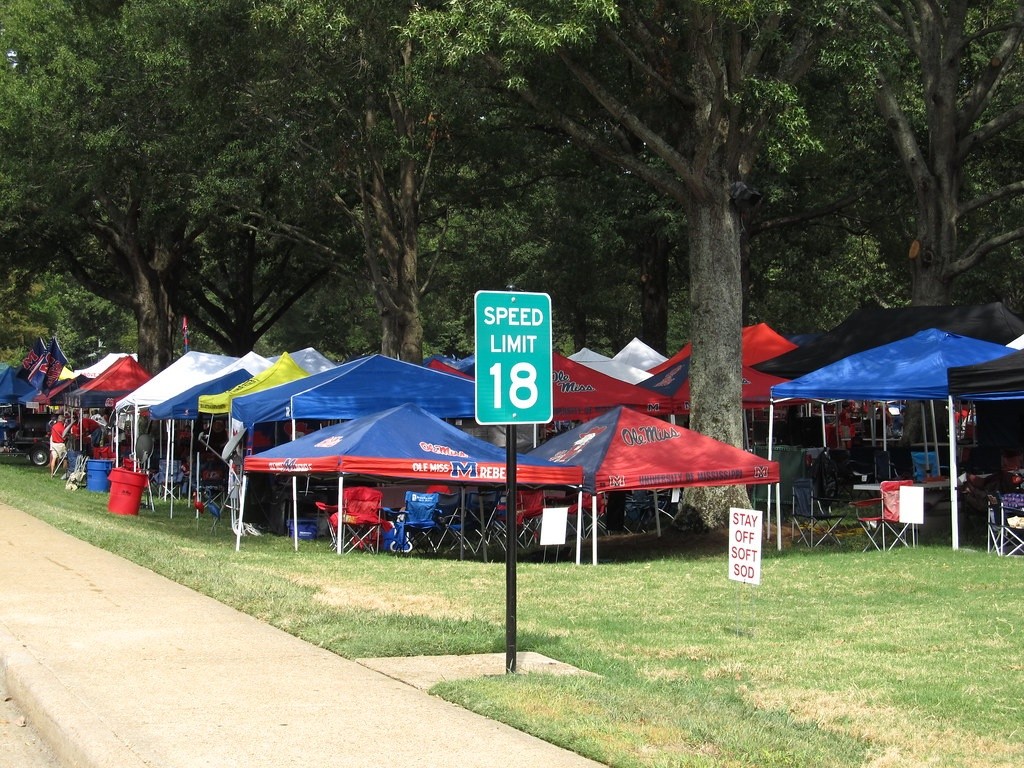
[956,400,970,426]
[879,406,893,437]
[892,407,906,439]
[199,418,228,461]
[48,407,126,471]
[786,405,801,420]
[965,434,1002,511]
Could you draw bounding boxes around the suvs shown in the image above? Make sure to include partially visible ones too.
[8,414,62,467]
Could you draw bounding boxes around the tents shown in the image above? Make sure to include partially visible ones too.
[0,353,115,464]
[67,303,1024,562]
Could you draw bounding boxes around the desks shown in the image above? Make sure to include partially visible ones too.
[975,468,1024,479]
[370,485,460,521]
[853,474,984,548]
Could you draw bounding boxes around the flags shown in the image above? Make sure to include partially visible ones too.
[16,336,68,397]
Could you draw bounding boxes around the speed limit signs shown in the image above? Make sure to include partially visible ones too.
[474,290,553,425]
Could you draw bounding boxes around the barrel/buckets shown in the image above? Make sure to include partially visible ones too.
[86,459,114,494]
[106,468,148,516]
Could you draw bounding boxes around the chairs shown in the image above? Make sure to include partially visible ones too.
[0,402,1024,564]
